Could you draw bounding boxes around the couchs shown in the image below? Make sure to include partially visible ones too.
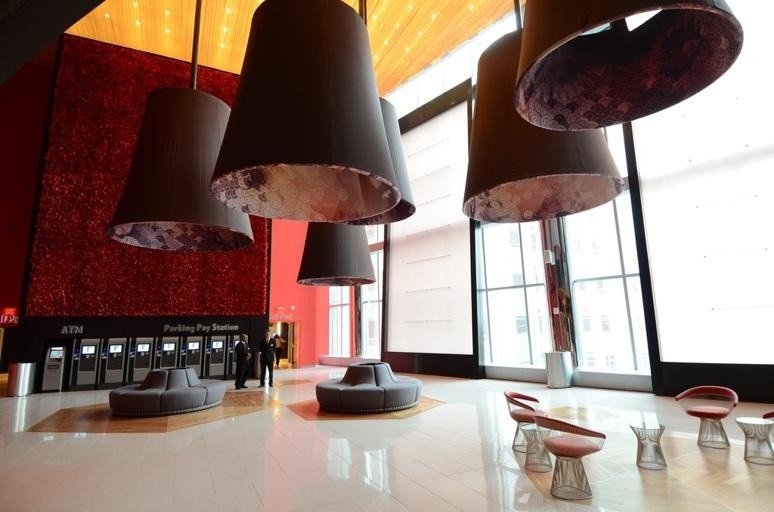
[316,362,423,413]
[109,366,225,416]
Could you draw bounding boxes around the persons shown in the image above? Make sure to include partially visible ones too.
[274,334,287,367]
[234,333,252,391]
[258,329,276,387]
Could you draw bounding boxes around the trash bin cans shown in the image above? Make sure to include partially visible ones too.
[544,352,573,389]
[7,359,38,397]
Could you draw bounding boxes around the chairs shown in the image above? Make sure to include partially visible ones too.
[503,389,540,454]
[533,416,606,501]
[674,386,738,449]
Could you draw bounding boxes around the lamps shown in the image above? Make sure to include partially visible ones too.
[513,1,744,133]
[104,0,255,254]
[295,222,376,286]
[461,1,626,224]
[343,0,417,226]
[209,0,401,224]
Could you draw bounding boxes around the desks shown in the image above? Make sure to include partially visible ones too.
[519,424,553,473]
[735,417,774,466]
[629,423,669,470]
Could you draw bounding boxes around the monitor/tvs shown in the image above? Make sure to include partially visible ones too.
[49,350,64,359]
[235,340,241,348]
[109,344,123,354]
[137,343,151,352]
[212,340,224,349]
[163,342,176,352]
[188,341,200,350]
[81,345,96,355]
